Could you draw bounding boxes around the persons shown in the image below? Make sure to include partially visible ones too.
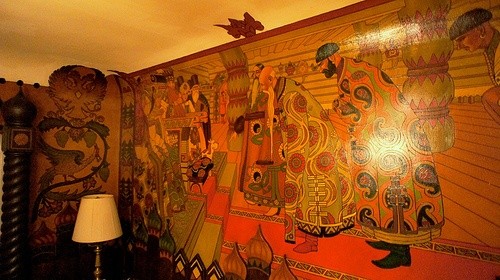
[449,7,500,124]
[237,62,291,217]
[259,65,357,253]
[132,62,213,211]
[315,42,445,268]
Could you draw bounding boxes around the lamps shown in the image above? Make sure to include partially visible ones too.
[71,193,123,280]
